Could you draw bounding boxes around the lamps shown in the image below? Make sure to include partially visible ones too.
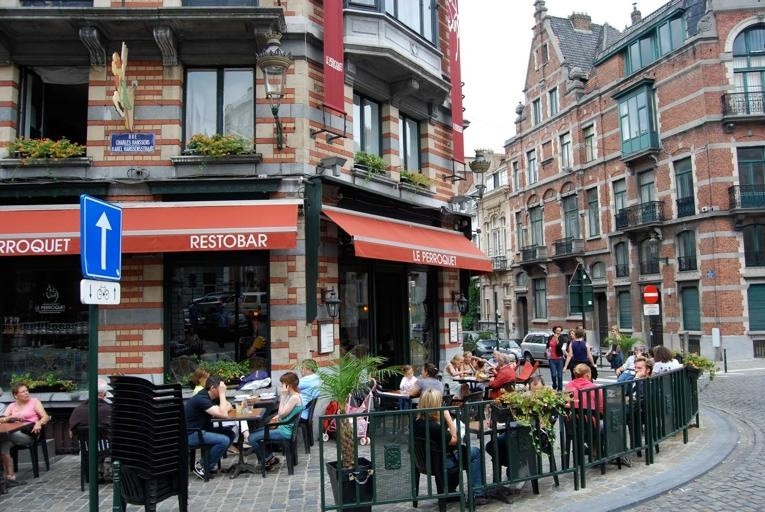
[451,290,468,316]
[320,285,343,318]
[467,159,491,198]
[254,47,295,149]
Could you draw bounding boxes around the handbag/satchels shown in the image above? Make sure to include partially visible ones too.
[544,349,552,360]
[608,351,613,363]
[238,370,271,391]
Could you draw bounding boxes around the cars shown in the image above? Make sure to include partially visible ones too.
[169,289,265,360]
[496,338,523,363]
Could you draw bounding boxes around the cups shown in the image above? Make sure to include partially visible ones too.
[472,368,492,379]
[234,396,255,414]
[483,404,492,424]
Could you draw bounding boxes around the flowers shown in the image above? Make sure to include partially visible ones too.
[1,134,86,184]
[189,132,245,160]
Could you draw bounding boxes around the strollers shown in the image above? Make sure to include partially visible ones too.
[321,376,379,448]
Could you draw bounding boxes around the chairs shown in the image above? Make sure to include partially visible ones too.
[76,374,190,512]
[256,409,301,478]
[443,359,539,417]
[413,421,468,512]
[561,407,621,475]
[9,424,50,479]
[299,398,317,454]
[186,428,221,482]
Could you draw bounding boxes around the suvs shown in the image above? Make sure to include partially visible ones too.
[520,331,599,366]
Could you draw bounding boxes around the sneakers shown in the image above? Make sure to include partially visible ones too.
[193,461,207,479]
[263,457,280,473]
[468,493,491,507]
[5,474,16,483]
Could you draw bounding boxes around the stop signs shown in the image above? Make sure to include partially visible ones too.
[642,284,659,304]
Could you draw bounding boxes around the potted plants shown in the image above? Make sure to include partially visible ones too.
[400,170,432,188]
[490,386,580,458]
[10,373,77,393]
[289,345,410,512]
[179,353,250,385]
[353,151,391,184]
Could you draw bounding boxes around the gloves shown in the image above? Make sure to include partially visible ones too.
[252,336,266,349]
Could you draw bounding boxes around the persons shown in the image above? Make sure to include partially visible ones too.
[182,373,236,482]
[217,296,271,382]
[191,367,251,456]
[398,323,686,505]
[257,358,322,429]
[0,383,50,480]
[247,372,304,472]
[68,377,113,453]
[0,386,6,417]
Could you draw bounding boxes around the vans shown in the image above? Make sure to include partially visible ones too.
[461,329,500,357]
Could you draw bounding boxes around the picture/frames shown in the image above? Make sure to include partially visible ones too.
[449,318,459,343]
[318,320,335,355]
